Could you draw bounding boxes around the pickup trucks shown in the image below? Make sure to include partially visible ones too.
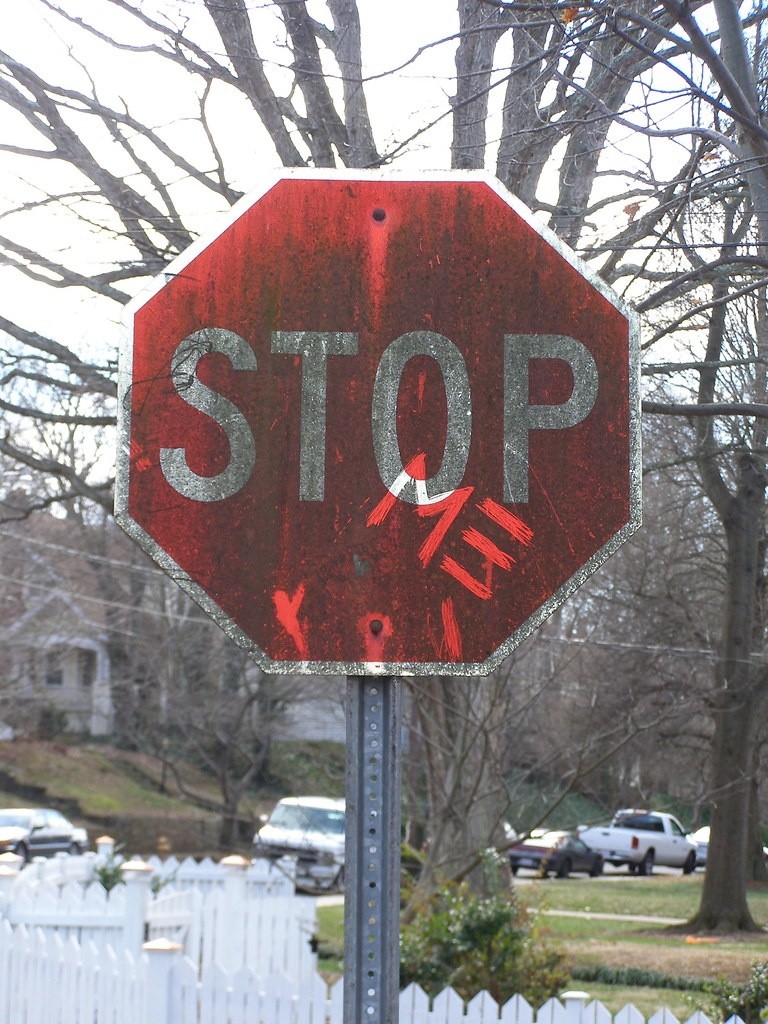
[575,807,697,876]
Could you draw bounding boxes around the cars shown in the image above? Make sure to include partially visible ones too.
[508,828,603,879]
[688,825,768,873]
[0,808,88,864]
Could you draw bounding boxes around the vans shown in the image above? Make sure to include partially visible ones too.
[251,797,346,895]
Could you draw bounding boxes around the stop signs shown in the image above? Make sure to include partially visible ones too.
[115,170,645,679]
